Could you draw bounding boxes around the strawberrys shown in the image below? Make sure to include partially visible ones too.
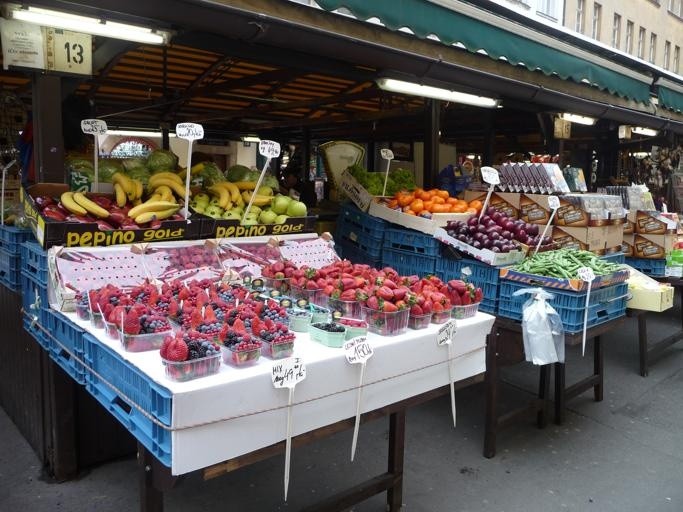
[166,246,218,272]
[225,312,230,323]
[265,319,273,330]
[323,277,362,317]
[191,309,203,331]
[90,289,99,313]
[255,305,261,314]
[183,299,193,314]
[124,308,140,335]
[357,277,409,335]
[109,306,122,329]
[231,245,281,264]
[271,304,280,313]
[251,317,268,337]
[104,303,115,321]
[292,264,333,303]
[263,259,295,284]
[162,283,169,295]
[331,259,449,290]
[168,339,188,362]
[160,336,173,359]
[196,290,209,309]
[449,278,482,318]
[268,299,276,310]
[431,287,451,324]
[175,331,183,341]
[205,305,216,321]
[411,289,434,331]
[149,290,157,308]
[220,324,229,343]
[178,288,190,301]
[232,318,246,334]
[99,289,111,313]
[169,301,180,315]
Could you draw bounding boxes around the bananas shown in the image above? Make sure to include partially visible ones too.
[178,161,205,180]
[146,172,193,198]
[206,181,244,212]
[60,184,110,218]
[243,187,273,207]
[128,200,185,224]
[235,181,257,188]
[109,172,143,207]
[147,186,177,204]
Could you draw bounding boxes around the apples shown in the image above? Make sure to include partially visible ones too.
[222,211,240,220]
[274,215,289,224]
[229,204,244,213]
[204,209,222,218]
[209,199,220,208]
[271,195,292,214]
[240,207,262,226]
[260,209,277,224]
[207,205,222,213]
[192,193,209,213]
[288,201,307,218]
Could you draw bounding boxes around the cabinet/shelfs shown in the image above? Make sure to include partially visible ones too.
[0,180,483,511]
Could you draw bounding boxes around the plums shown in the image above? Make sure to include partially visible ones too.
[447,206,523,251]
[485,203,554,248]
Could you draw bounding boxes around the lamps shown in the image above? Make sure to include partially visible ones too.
[556,111,597,126]
[630,125,658,137]
[0,0,180,50]
[373,70,503,108]
[98,126,177,139]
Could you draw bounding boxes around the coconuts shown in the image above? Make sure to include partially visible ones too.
[99,166,119,180]
[97,159,118,167]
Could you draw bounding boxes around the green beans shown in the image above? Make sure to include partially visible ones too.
[507,249,623,280]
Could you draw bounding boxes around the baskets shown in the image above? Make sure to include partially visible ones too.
[336,205,437,280]
[1,227,49,348]
[433,256,663,335]
[48,310,171,468]
